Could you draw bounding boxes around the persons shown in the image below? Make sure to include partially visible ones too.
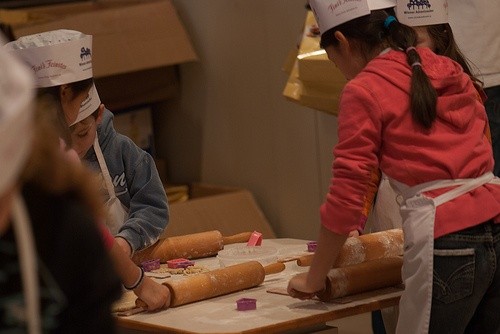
[286,1,500,334]
[1,27,172,334]
[395,0,485,89]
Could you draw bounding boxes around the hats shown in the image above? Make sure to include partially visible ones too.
[393,1,450,26]
[2,29,93,85]
[2,48,37,189]
[306,0,398,36]
[63,82,102,126]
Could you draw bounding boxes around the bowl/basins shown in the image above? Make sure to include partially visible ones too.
[216,245,280,269]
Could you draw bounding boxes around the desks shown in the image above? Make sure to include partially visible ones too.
[113,238,404,334]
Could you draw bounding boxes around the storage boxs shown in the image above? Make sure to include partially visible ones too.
[10,1,200,114]
